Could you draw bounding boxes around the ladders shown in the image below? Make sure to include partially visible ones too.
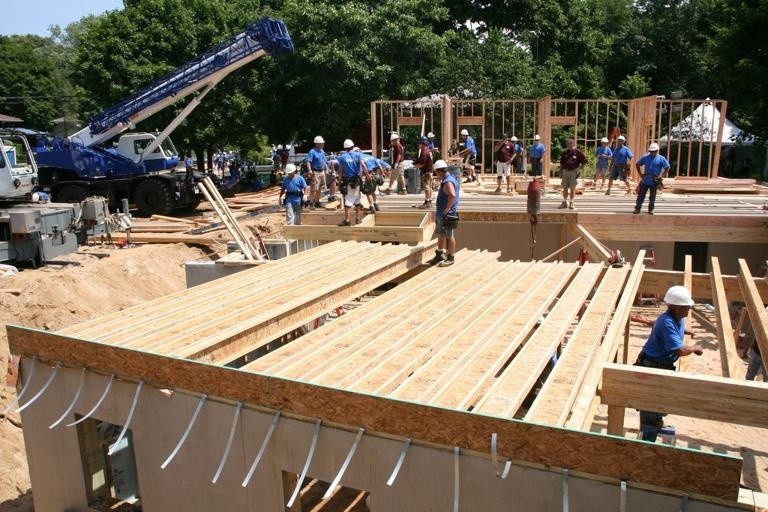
[639,247,660,308]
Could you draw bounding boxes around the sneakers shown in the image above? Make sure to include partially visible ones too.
[439,254,454,266]
[430,250,445,264]
[365,204,378,215]
[559,201,566,209]
[356,218,362,223]
[310,202,323,209]
[338,221,352,226]
[570,202,575,208]
[420,201,431,208]
[380,189,390,195]
[464,177,476,183]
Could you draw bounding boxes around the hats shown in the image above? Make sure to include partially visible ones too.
[534,135,540,141]
[511,136,518,141]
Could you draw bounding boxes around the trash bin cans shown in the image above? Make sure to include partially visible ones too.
[448,166,461,190]
[407,167,422,193]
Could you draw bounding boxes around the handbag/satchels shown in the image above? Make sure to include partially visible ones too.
[443,216,458,229]
[655,177,664,190]
[623,164,631,177]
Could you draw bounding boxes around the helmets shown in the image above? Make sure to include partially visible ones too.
[285,164,296,174]
[314,136,324,143]
[427,133,434,138]
[618,135,626,141]
[648,143,660,151]
[601,138,608,143]
[390,134,399,140]
[434,160,448,170]
[664,285,694,307]
[461,129,469,135]
[344,139,354,148]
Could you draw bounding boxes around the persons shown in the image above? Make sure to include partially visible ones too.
[634,143,670,214]
[270,144,289,175]
[429,160,460,266]
[279,164,307,226]
[590,136,634,195]
[745,338,768,382]
[185,157,192,172]
[494,134,523,193]
[558,138,588,208]
[382,134,407,196]
[633,285,703,442]
[306,136,385,226]
[459,129,476,183]
[212,150,235,167]
[530,135,545,177]
[415,132,438,209]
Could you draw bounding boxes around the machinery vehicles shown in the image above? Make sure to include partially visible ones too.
[0,126,86,269]
[8,16,296,220]
